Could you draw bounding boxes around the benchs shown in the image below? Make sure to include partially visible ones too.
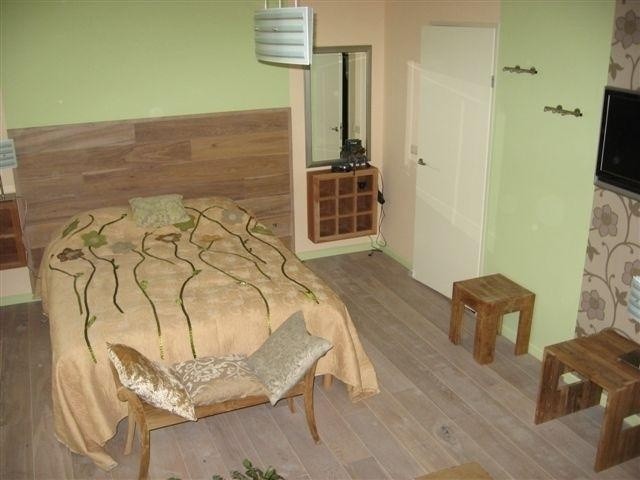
[108,357,322,480]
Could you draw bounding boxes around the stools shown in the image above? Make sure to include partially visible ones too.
[534,329,640,474]
[448,273,536,365]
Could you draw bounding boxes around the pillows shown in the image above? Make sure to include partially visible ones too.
[127,193,191,229]
[105,311,333,421]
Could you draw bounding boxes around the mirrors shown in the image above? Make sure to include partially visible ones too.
[305,45,372,168]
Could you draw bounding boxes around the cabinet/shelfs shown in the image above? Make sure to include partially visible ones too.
[0,192,28,271]
[306,165,380,245]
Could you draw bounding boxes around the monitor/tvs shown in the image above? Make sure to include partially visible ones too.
[592,86,640,201]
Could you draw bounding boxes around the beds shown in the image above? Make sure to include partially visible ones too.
[39,195,345,421]
